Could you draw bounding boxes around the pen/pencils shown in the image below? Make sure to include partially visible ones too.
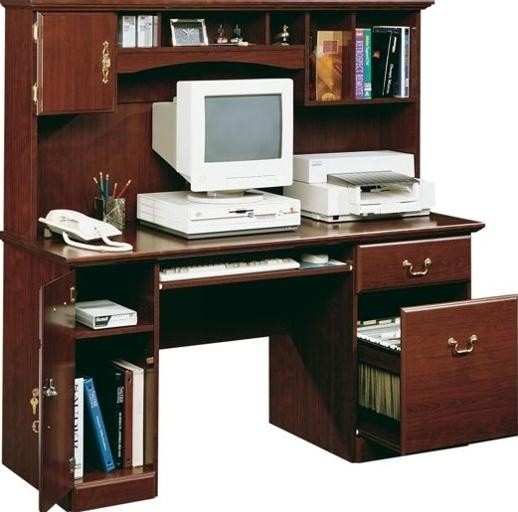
[93,172,133,198]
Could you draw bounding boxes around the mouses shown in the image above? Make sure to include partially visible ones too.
[301,252,330,265]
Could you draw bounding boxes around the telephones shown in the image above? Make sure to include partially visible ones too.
[38,209,123,243]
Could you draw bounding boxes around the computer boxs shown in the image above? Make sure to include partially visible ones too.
[136,187,301,240]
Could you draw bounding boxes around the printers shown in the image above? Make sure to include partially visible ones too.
[283,149,430,222]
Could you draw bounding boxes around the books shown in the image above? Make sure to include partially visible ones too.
[74,357,157,481]
[309,26,410,102]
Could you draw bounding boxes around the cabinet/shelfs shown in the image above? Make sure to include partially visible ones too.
[0,0,518,512]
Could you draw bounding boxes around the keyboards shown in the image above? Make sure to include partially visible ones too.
[158,255,301,283]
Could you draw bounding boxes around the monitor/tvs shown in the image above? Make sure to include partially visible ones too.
[152,78,295,204]
